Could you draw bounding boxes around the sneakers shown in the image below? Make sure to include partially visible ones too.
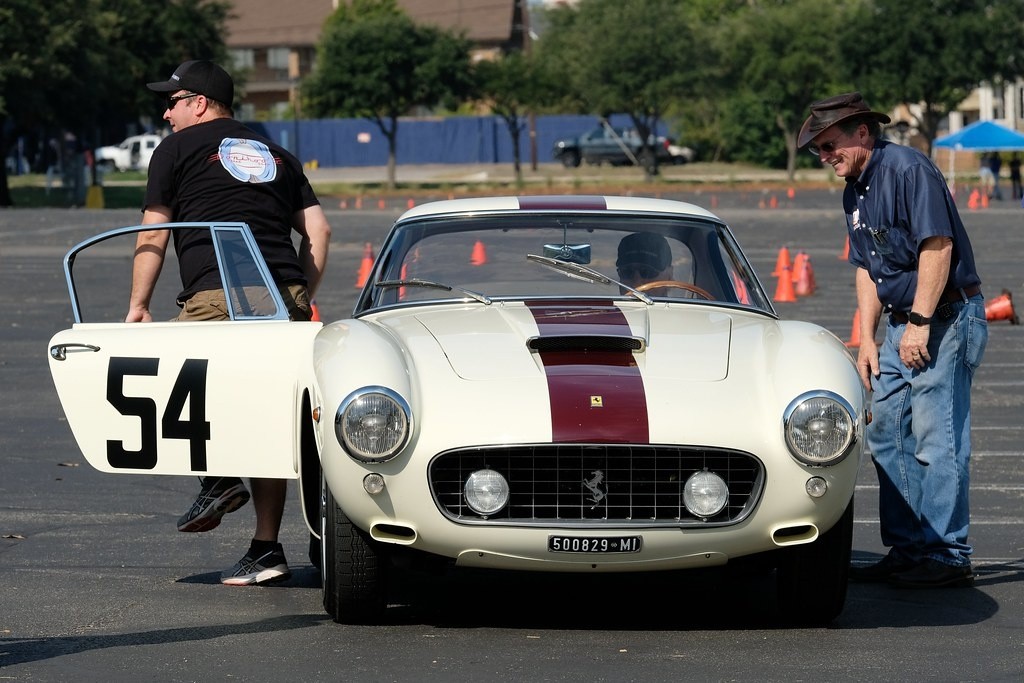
[176,476,250,533]
[220,539,289,585]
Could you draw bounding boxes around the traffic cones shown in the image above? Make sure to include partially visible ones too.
[983,288,1018,326]
[354,240,377,286]
[470,238,487,266]
[844,306,861,346]
[772,243,812,305]
[335,195,425,210]
[968,188,988,208]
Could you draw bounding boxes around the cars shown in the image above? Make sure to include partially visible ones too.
[46,192,863,629]
[96,136,167,172]
[551,126,697,170]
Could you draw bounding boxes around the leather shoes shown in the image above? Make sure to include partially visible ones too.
[889,557,974,588]
[850,551,919,581]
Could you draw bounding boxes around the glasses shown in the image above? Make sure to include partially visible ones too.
[167,93,197,111]
[617,266,660,279]
[808,130,845,155]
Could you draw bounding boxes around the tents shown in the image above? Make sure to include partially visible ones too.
[932,120,1024,197]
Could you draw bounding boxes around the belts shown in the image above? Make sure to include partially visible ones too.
[897,284,981,324]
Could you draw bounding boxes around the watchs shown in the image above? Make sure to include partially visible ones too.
[908,312,931,326]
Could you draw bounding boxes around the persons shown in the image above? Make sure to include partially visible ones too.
[978,151,1024,201]
[124,60,331,585]
[10,128,93,210]
[615,233,674,296]
[798,92,988,589]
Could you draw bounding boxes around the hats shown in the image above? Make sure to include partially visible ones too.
[617,233,672,272]
[797,92,891,150]
[147,60,234,108]
